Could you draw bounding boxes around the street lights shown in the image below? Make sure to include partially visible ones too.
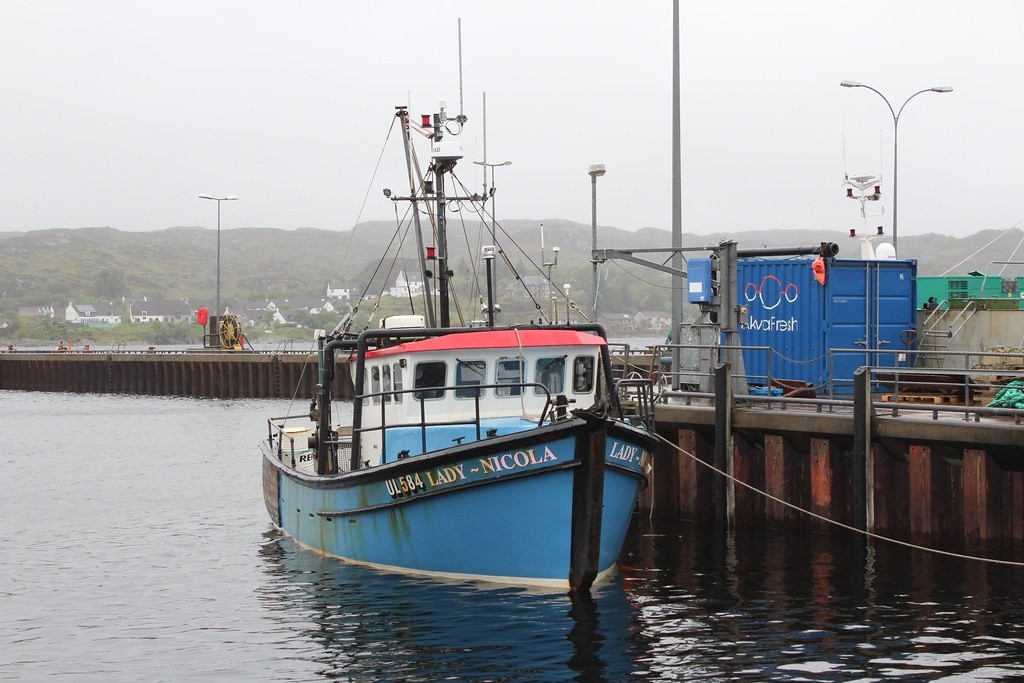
[840,80,954,260]
[200,194,240,347]
[589,164,606,322]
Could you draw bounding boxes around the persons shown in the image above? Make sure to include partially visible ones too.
[58,340,67,351]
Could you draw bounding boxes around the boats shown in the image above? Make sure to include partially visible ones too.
[255,16,663,597]
